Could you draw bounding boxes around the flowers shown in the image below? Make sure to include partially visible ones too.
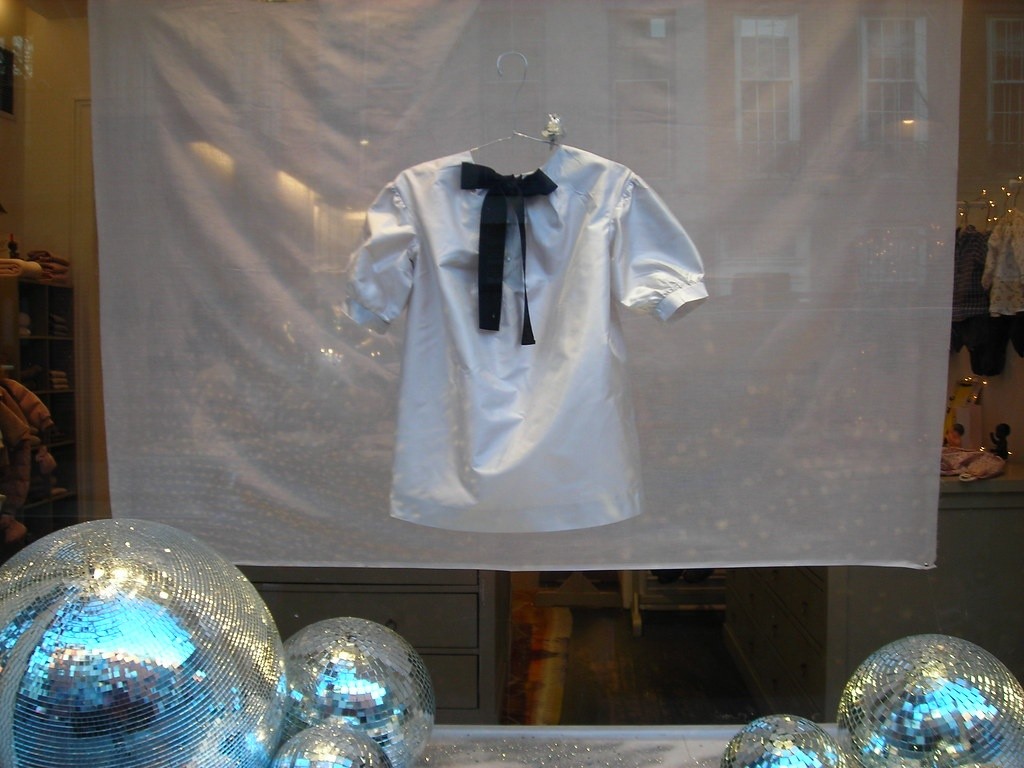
[541,111,566,151]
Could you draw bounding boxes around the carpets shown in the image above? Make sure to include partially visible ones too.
[510,606,572,725]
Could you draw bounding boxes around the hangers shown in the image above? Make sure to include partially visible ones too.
[957,175,1024,236]
[469,51,562,154]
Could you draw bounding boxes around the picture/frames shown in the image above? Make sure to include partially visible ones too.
[0,41,17,120]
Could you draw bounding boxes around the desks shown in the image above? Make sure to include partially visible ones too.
[410,722,843,768]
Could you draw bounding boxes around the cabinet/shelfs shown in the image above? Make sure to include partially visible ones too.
[722,492,1024,722]
[233,565,511,725]
[1,275,79,561]
